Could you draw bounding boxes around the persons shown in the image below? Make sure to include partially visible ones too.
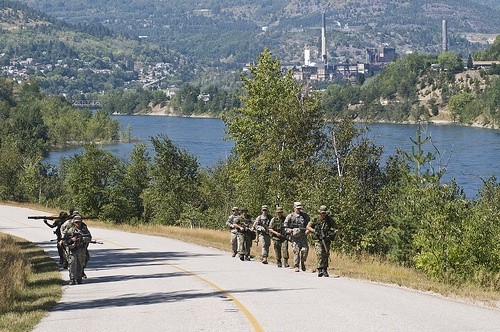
[284,202,310,272]
[269,207,290,267]
[226,207,240,257]
[44,208,91,284]
[307,205,339,277]
[233,208,255,260]
[254,205,273,264]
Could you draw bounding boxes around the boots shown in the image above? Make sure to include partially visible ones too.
[318,268,329,277]
[284,259,289,267]
[294,263,299,272]
[301,261,306,271]
[277,260,282,267]
[262,256,268,264]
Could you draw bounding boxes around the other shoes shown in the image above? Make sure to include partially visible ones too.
[69,279,75,285]
[240,254,244,261]
[232,253,236,257]
[83,273,87,279]
[245,257,251,261]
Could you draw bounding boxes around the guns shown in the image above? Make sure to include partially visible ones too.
[236,222,253,232]
[50,238,65,241]
[315,225,332,262]
[254,222,260,246]
[70,234,103,244]
[272,233,292,242]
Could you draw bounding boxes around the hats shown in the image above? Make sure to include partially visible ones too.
[262,205,269,210]
[241,207,248,211]
[294,202,302,208]
[232,207,239,210]
[318,205,329,212]
[275,207,283,212]
[74,215,81,221]
[73,211,79,216]
